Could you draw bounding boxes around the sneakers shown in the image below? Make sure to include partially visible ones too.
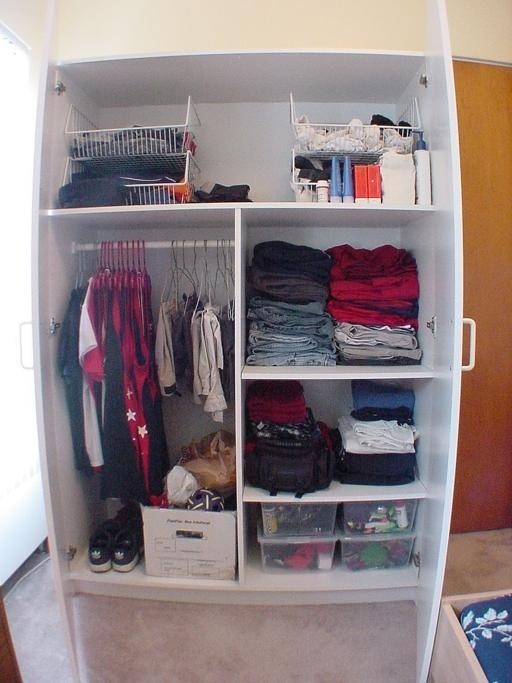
[87,516,114,572]
[113,522,142,573]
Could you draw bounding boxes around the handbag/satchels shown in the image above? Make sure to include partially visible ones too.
[246,436,335,492]
[180,429,236,498]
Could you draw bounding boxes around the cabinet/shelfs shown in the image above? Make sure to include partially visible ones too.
[29,0,477,683]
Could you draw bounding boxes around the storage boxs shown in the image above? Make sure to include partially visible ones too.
[138,496,236,581]
[428,589,512,683]
[256,499,416,575]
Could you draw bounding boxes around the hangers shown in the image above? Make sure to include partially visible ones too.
[72,237,238,326]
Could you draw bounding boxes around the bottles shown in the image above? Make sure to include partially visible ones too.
[316,180,328,202]
